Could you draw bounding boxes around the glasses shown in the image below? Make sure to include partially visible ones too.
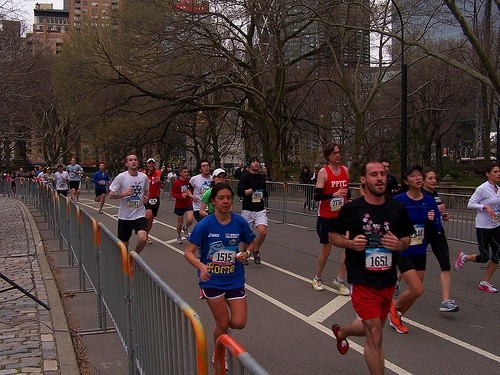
[201,164,209,167]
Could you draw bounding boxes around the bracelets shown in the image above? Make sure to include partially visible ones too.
[245,249,251,258]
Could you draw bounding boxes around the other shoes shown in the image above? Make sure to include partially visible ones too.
[146,236,151,244]
[177,226,189,244]
[98,209,103,213]
[267,210,270,213]
[301,207,310,214]
[212,349,229,370]
[311,207,318,211]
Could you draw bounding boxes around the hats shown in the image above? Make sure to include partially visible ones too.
[57,164,64,169]
[147,158,156,163]
[260,163,265,167]
[212,168,225,179]
[47,167,50,170]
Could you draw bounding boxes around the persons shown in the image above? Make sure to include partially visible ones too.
[333,184,366,295]
[388,166,440,333]
[0,157,83,202]
[92,163,111,214]
[329,161,417,375]
[299,166,320,211]
[263,165,273,213]
[108,153,149,253]
[394,167,459,311]
[159,168,178,194]
[312,143,350,295]
[380,159,399,195]
[186,160,213,222]
[183,183,256,370]
[172,168,194,244]
[141,158,163,245]
[238,157,268,265]
[199,169,235,299]
[454,164,500,293]
[235,163,251,201]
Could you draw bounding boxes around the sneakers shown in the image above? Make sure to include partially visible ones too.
[389,298,403,318]
[242,259,250,265]
[453,251,468,272]
[440,299,460,312]
[254,250,261,264]
[312,275,324,290]
[394,281,400,297]
[332,277,351,296]
[332,324,349,356]
[479,280,498,293]
[389,315,409,335]
